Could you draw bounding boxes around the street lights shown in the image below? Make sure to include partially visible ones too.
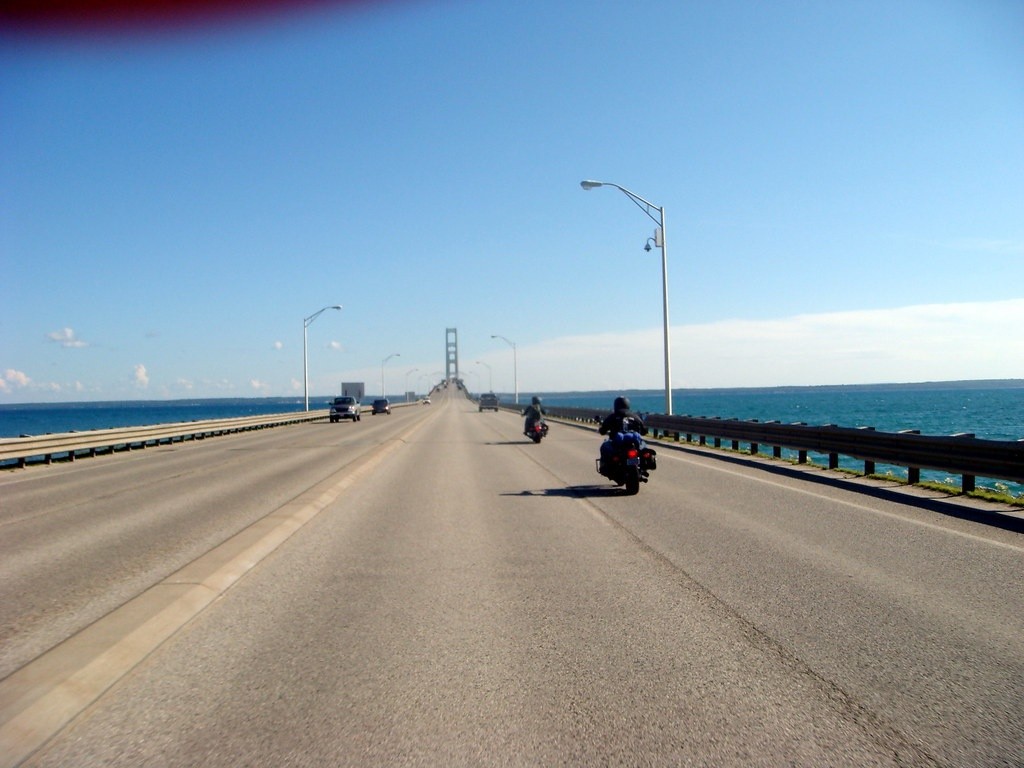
[581,179,672,416]
[492,335,519,403]
[303,305,342,411]
[381,353,400,399]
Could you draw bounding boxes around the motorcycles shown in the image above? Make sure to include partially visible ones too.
[592,415,658,495]
[521,409,549,444]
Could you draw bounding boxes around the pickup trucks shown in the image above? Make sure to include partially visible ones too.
[329,397,362,422]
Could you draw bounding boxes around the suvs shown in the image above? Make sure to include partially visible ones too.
[478,394,499,412]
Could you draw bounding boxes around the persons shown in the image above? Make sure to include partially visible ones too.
[598,395,647,473]
[523,396,548,433]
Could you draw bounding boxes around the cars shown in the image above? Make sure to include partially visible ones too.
[423,397,430,403]
[370,399,391,414]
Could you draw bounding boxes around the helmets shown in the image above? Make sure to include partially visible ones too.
[532,396,541,404]
[614,397,630,411]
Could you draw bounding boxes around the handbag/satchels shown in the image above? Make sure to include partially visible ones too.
[616,431,641,444]
